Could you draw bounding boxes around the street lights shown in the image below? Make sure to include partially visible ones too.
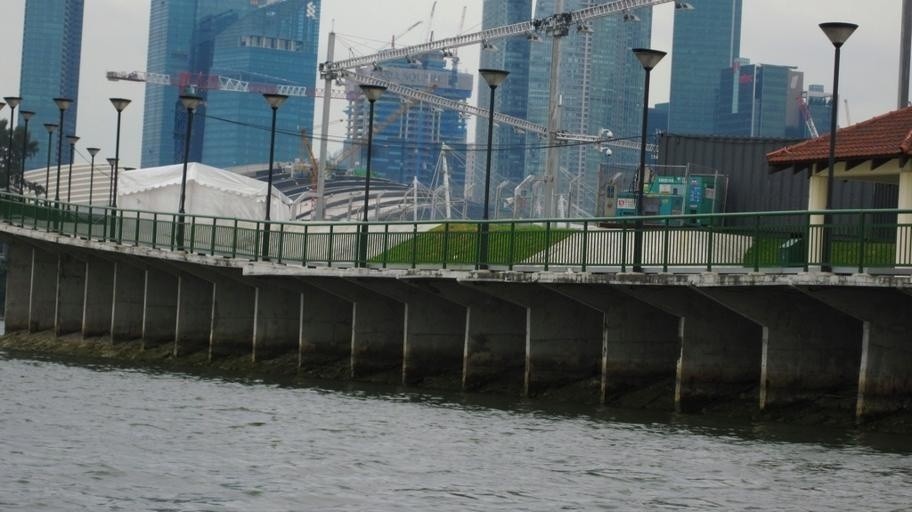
[478,68,510,270]
[822,22,859,273]
[177,94,204,250]
[355,84,386,268]
[632,48,667,273]
[261,93,288,261]
[0,96,132,242]
